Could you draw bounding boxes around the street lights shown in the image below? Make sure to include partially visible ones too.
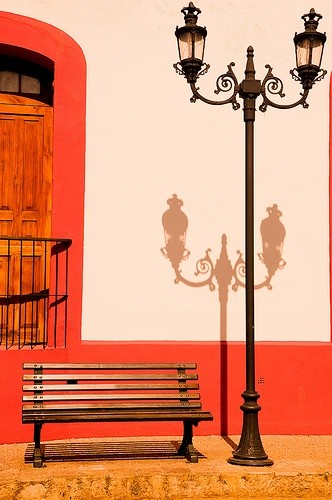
[173,1,328,466]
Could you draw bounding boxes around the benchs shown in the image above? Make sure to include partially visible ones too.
[21,361,213,469]
